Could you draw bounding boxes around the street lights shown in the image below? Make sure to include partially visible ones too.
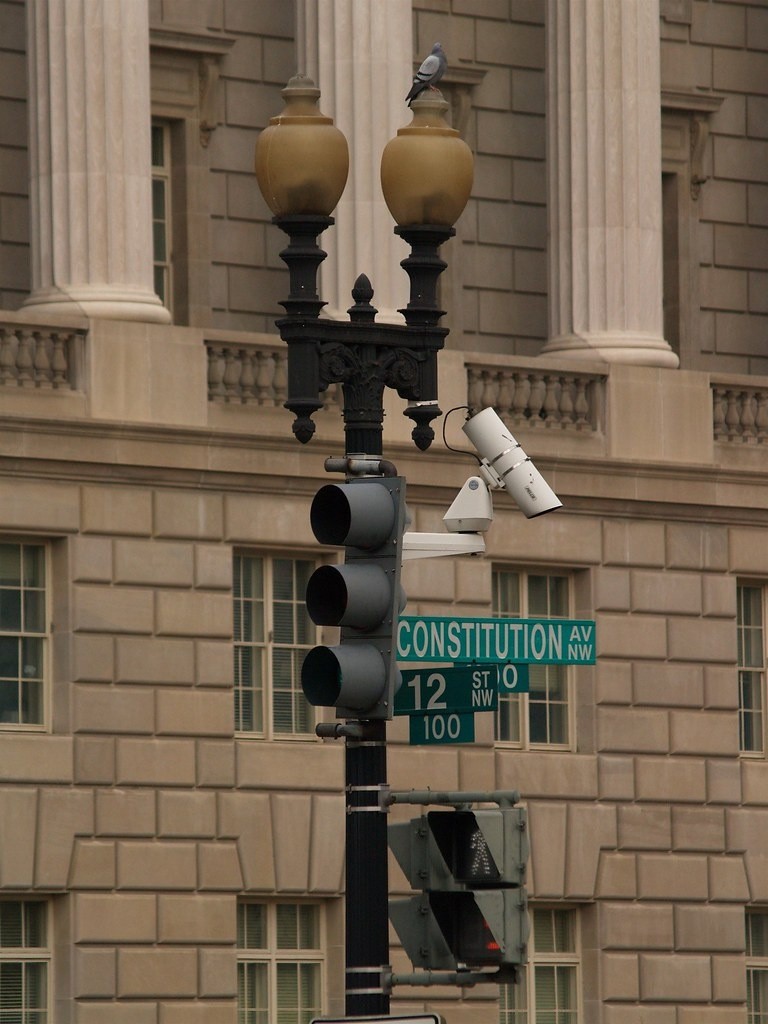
[251,70,563,1024]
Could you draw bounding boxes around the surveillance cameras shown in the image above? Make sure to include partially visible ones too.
[463,406,565,522]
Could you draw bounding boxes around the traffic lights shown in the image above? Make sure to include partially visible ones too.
[302,476,402,714]
[422,791,526,980]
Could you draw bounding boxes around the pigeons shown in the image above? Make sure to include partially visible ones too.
[405,42,449,108]
